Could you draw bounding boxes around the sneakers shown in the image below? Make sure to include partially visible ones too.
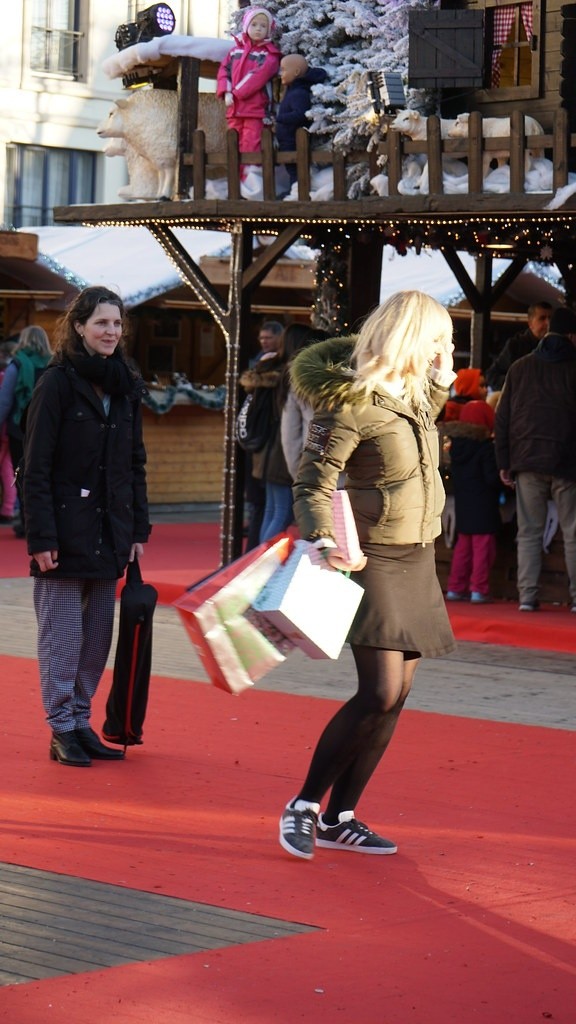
[314,811,398,854]
[279,796,321,859]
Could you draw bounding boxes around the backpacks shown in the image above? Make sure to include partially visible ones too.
[235,385,277,452]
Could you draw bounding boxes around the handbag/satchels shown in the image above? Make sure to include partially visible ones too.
[173,528,299,696]
[255,539,364,659]
[100,556,158,747]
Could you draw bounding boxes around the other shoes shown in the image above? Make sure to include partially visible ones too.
[445,591,467,598]
[520,602,542,611]
[469,591,492,602]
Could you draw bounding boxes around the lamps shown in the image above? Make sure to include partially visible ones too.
[114,3,175,52]
[121,64,161,90]
[367,69,406,115]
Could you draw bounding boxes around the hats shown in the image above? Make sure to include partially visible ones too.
[236,5,275,43]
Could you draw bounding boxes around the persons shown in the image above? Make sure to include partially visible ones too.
[0,326,54,536]
[279,293,459,856]
[271,52,328,198]
[20,286,152,766]
[215,7,279,182]
[236,317,374,557]
[436,304,576,612]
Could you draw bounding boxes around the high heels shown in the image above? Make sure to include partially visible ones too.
[49,732,93,766]
[76,729,123,760]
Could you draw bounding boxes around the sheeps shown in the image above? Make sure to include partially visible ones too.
[389,108,469,187]
[446,112,544,174]
[97,90,230,201]
